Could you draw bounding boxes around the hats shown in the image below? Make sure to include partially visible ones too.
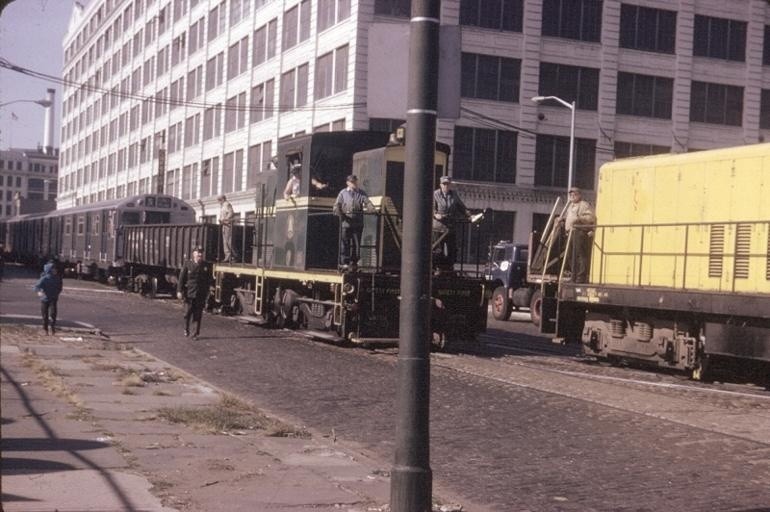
[441,176,451,184]
[347,175,358,181]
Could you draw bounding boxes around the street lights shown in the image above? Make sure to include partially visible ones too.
[532,96,576,206]
[0,97,53,111]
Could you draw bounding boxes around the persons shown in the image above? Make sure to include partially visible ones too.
[331,174,374,272]
[432,175,473,271]
[35,263,61,336]
[176,246,213,342]
[217,193,240,263]
[553,186,595,283]
[282,164,330,201]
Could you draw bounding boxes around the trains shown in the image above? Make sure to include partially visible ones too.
[541,143,769,382]
[0,126,491,352]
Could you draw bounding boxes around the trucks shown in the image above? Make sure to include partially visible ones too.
[483,239,545,325]
